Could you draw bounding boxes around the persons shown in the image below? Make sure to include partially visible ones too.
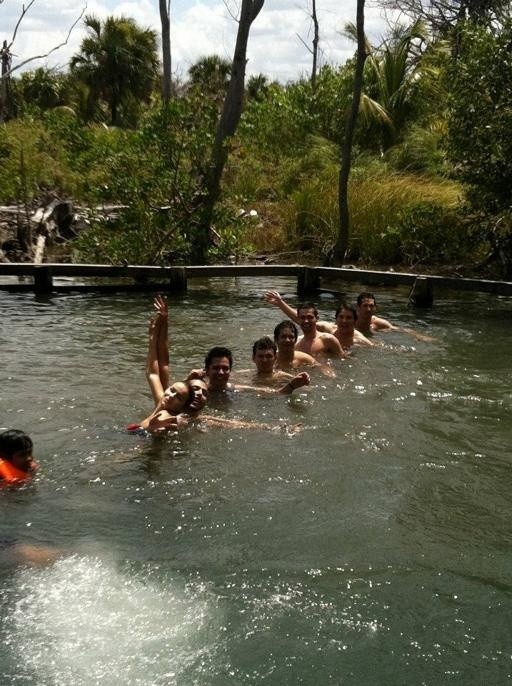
[0,429,39,491]
[265,291,393,371]
[127,294,311,440]
